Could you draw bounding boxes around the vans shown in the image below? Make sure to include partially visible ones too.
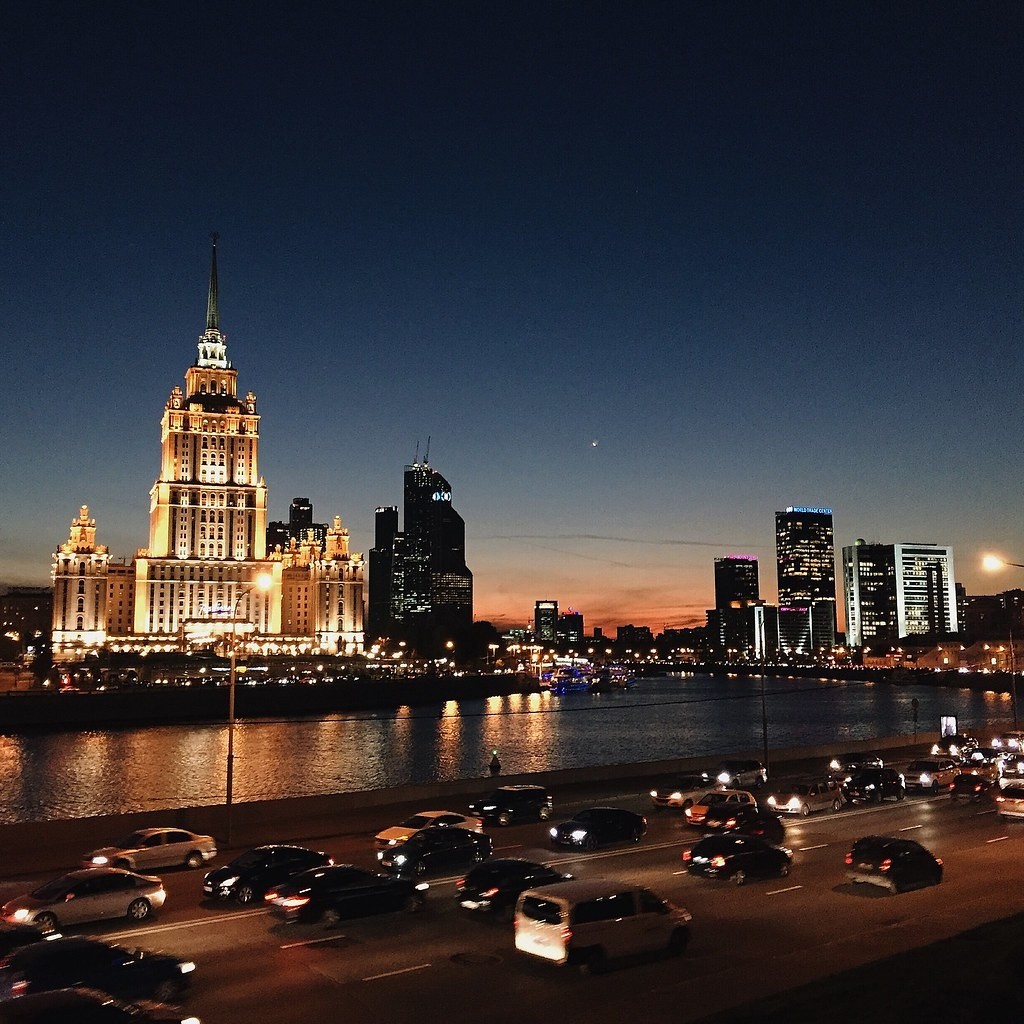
[513,879,695,974]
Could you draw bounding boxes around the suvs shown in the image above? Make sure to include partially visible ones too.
[468,785,553,827]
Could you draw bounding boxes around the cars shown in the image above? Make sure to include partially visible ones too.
[376,825,495,881]
[0,866,168,937]
[454,859,577,923]
[650,774,724,809]
[701,801,757,836]
[828,730,1024,806]
[686,790,756,827]
[0,987,206,1024]
[682,834,794,886]
[262,864,427,931]
[704,758,769,789]
[764,775,846,821]
[201,844,335,905]
[0,935,195,1005]
[373,809,484,853]
[81,827,218,874]
[997,780,1024,820]
[845,833,944,894]
[549,806,649,852]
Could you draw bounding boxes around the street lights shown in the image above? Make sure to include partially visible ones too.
[222,573,274,856]
